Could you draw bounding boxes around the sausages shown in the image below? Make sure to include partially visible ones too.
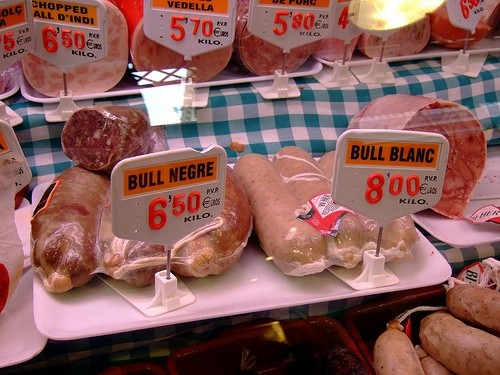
[30,94,499,375]
